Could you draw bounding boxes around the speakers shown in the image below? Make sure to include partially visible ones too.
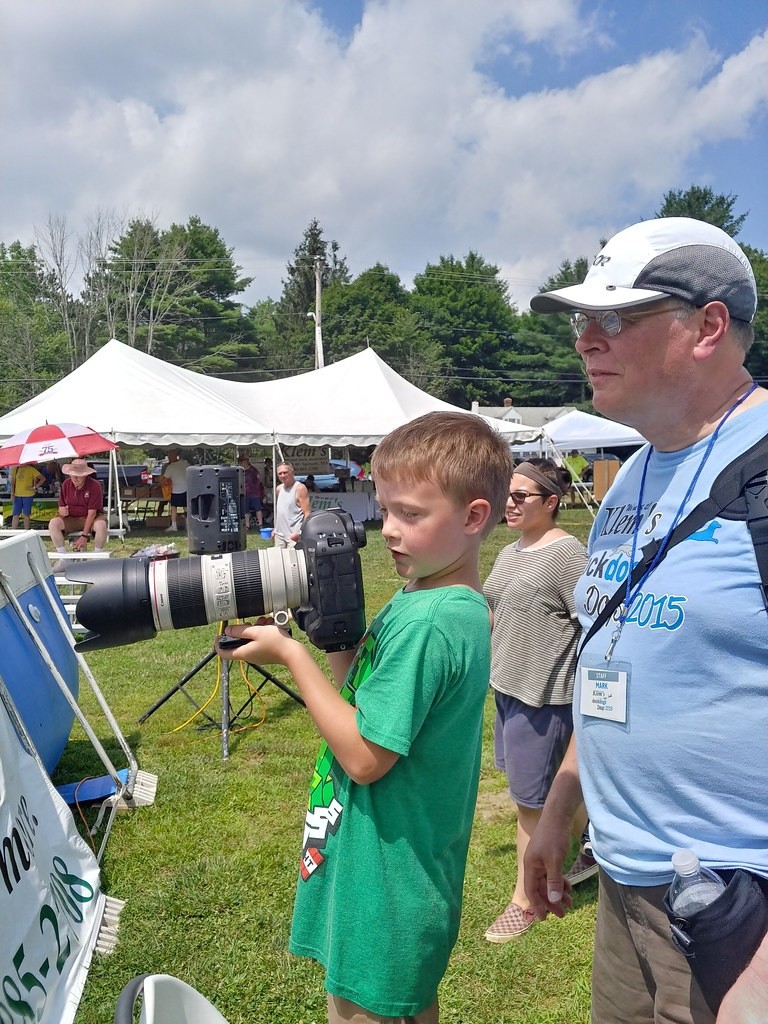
[187,465,247,555]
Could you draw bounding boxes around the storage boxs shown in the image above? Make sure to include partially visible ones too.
[345,481,354,493]
[354,481,373,492]
[147,516,170,527]
[121,487,135,497]
[136,487,150,498]
[151,487,163,497]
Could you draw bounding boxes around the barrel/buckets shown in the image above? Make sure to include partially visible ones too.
[260,528,273,540]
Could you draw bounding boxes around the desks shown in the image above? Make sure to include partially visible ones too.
[309,493,368,521]
[106,497,171,522]
[559,482,593,511]
[370,490,382,521]
[0,498,58,501]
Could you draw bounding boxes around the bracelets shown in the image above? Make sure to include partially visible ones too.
[35,485,38,488]
[11,493,14,495]
[81,534,88,538]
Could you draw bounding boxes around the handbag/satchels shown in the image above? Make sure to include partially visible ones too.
[662,865,768,1017]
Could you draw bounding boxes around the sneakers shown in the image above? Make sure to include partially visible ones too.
[484,901,550,944]
[563,844,599,887]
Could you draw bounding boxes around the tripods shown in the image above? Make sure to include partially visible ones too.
[136,620,307,762]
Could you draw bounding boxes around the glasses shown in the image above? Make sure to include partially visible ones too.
[509,492,550,504]
[569,307,685,340]
[277,462,291,467]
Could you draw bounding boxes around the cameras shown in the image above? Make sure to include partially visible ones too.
[64,508,367,654]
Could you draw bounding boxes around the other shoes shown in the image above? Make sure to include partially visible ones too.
[164,525,178,532]
[52,559,73,572]
[258,525,264,533]
[247,524,251,531]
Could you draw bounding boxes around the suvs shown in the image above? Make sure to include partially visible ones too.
[293,459,365,490]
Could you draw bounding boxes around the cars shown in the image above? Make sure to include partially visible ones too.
[582,453,624,491]
[91,464,148,487]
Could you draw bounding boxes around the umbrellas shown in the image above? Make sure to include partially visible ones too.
[0,419,120,506]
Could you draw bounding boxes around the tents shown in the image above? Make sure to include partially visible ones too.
[508,410,648,510]
[0,338,544,543]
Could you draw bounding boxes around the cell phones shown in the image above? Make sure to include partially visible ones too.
[219,627,292,649]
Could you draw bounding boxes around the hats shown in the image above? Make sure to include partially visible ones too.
[571,450,578,454]
[265,458,271,463]
[529,216,758,324]
[237,453,249,460]
[61,459,97,476]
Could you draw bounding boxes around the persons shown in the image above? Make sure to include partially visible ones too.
[237,454,265,532]
[482,458,599,943]
[41,461,62,491]
[562,450,589,507]
[9,465,46,530]
[304,475,321,492]
[271,461,311,549]
[164,449,188,531]
[157,461,170,516]
[523,217,768,1024]
[49,459,108,573]
[214,410,514,1024]
[264,458,273,488]
